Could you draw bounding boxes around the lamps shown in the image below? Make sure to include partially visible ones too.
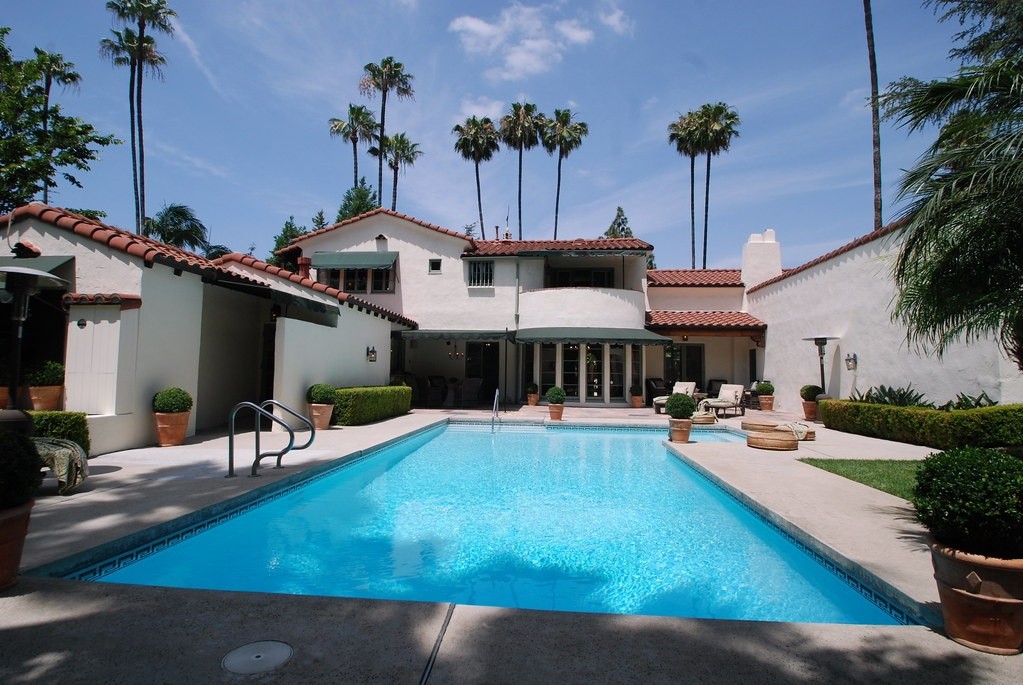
[845,353,857,370]
[366,346,377,362]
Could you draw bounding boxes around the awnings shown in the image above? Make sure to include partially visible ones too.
[514,327,673,346]
[0,256,75,274]
[309,251,399,280]
[389,330,517,344]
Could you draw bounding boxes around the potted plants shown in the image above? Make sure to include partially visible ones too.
[546,387,566,421]
[152,387,193,446]
[630,385,643,408]
[27,361,65,411]
[0,366,25,410]
[800,385,823,420]
[526,382,538,406]
[758,383,775,410]
[910,445,1023,655]
[306,383,337,430]
[665,392,696,443]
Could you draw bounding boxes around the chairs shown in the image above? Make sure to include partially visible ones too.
[647,377,770,419]
